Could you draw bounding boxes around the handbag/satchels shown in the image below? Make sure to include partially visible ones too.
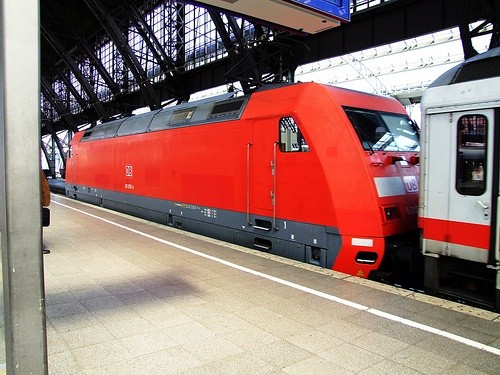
[43,207,51,227]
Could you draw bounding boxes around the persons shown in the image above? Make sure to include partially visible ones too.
[41,170,50,254]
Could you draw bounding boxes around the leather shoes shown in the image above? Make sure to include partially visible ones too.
[42,249,51,254]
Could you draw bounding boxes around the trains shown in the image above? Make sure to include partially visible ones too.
[66,47,500,314]
